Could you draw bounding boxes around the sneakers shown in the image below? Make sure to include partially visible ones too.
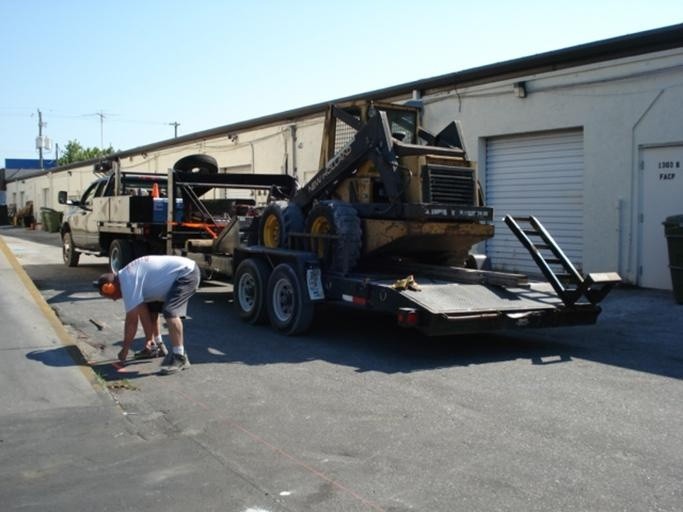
[160,351,191,375]
[134,342,168,359]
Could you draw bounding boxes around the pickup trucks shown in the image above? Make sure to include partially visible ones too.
[58,171,256,275]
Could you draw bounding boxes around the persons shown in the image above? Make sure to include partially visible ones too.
[97,254,201,373]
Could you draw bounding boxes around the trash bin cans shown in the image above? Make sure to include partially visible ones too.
[40,207,63,232]
[662,214,683,305]
